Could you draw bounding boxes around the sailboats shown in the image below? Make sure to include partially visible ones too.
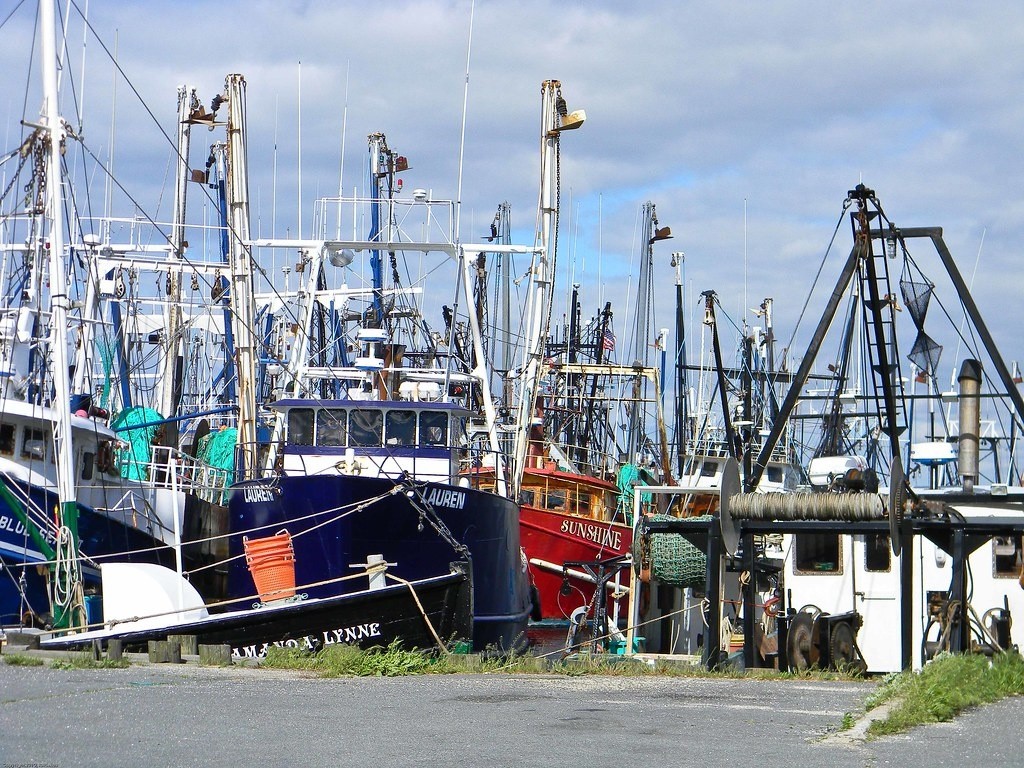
[1,0,1024,676]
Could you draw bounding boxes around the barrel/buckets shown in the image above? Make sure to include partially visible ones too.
[243,529,296,602]
